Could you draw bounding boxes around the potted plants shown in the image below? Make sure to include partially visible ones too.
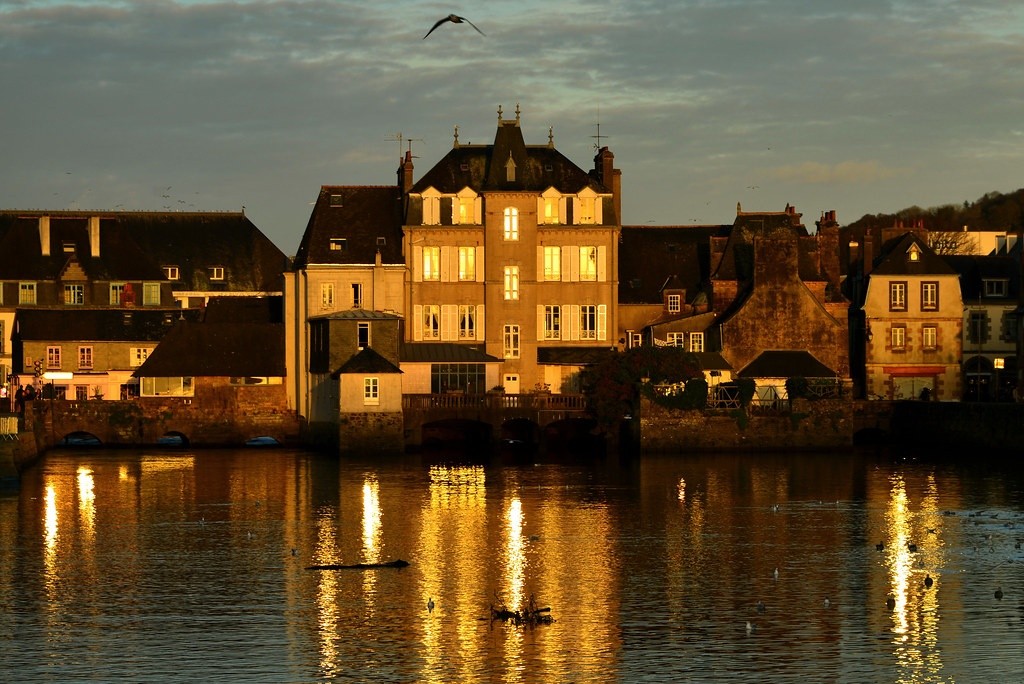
[491,385,505,395]
[446,383,463,393]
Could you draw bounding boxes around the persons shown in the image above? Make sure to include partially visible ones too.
[0,385,41,412]
[656,377,686,396]
[962,378,1024,403]
[891,385,930,401]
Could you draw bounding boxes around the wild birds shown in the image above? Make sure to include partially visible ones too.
[291,548,297,555]
[428,597,434,608]
[529,534,540,541]
[198,514,206,524]
[420,13,488,41]
[248,531,255,538]
[745,500,1024,631]
